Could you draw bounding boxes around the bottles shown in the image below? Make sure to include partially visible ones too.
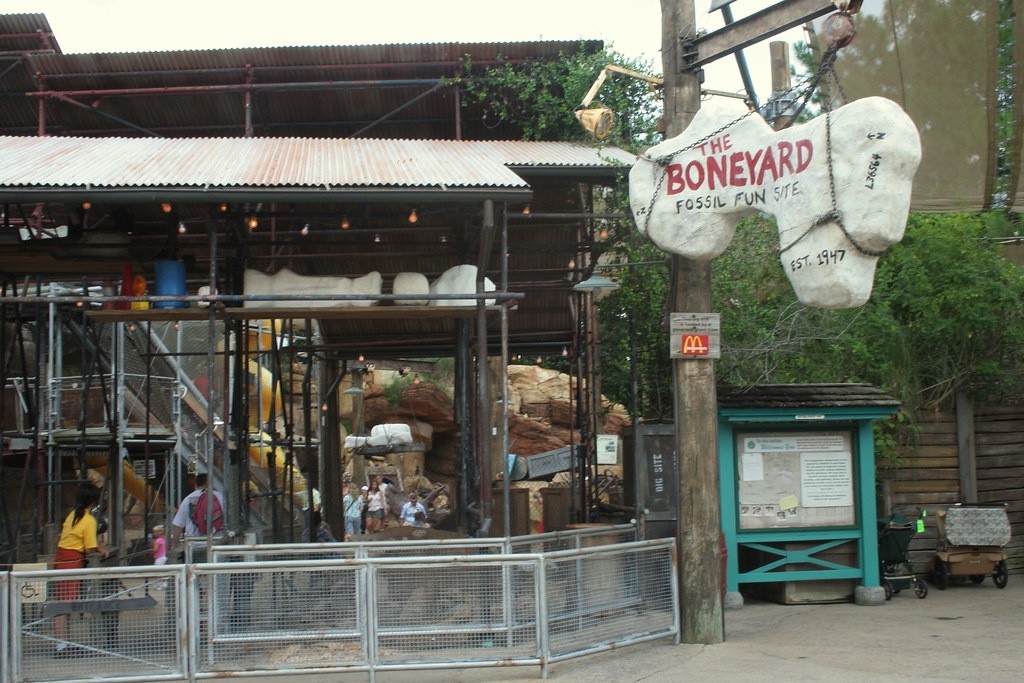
[119,263,133,310]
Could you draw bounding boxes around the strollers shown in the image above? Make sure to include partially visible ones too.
[877,507,929,601]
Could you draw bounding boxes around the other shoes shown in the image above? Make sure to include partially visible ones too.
[55,647,91,659]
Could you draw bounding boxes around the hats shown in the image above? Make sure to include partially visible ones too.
[361,486,369,490]
[409,493,418,502]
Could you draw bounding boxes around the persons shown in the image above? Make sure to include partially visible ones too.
[169,472,224,551]
[151,524,169,592]
[301,474,431,543]
[51,483,110,660]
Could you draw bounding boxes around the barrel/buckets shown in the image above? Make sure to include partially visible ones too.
[153,260,189,308]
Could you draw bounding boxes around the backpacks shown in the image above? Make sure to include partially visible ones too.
[189,489,223,535]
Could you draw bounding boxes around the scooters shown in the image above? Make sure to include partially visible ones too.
[931,502,1011,590]
